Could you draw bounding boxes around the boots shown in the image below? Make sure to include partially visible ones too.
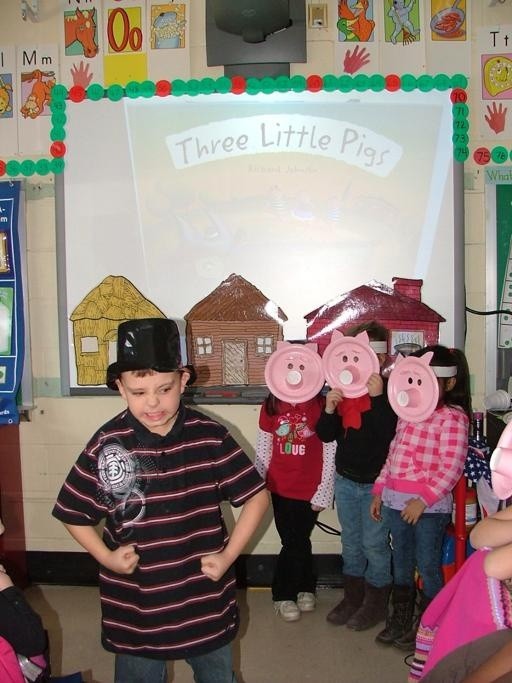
[327,575,367,625]
[348,583,391,631]
[392,593,431,650]
[375,585,416,642]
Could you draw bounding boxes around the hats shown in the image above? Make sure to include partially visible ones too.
[105,318,200,390]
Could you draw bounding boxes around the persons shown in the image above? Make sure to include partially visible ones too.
[254,390,338,623]
[51,321,270,683]
[315,377,396,633]
[1,522,82,683]
[370,347,474,650]
[403,506,512,683]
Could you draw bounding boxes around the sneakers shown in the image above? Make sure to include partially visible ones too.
[274,599,301,623]
[299,592,316,613]
